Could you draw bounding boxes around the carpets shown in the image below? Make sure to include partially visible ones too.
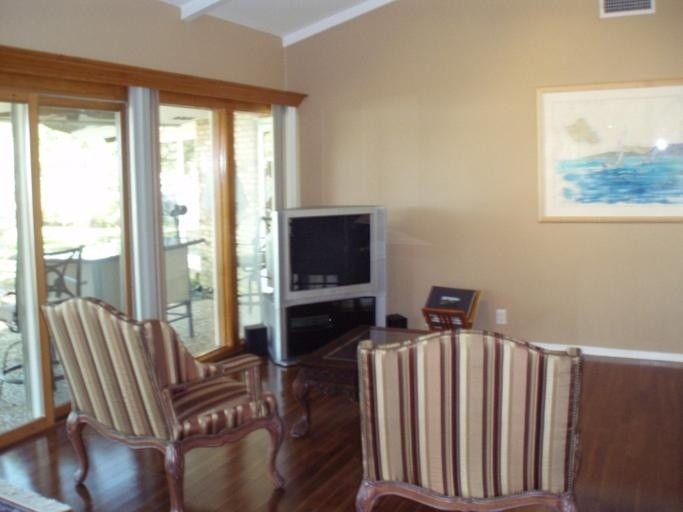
[0,477,73,512]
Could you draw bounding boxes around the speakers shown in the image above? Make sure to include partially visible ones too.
[244,324,268,355]
[385,314,407,328]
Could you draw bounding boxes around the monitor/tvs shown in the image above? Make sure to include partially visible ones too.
[272,207,387,294]
[279,292,387,363]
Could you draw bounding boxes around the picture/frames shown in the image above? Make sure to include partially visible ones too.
[536,77,683,222]
[599,0,655,18]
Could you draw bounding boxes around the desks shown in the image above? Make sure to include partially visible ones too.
[43,237,205,337]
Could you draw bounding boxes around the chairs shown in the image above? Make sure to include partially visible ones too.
[423,310,468,331]
[0,246,86,401]
[356,331,582,512]
[39,297,285,512]
